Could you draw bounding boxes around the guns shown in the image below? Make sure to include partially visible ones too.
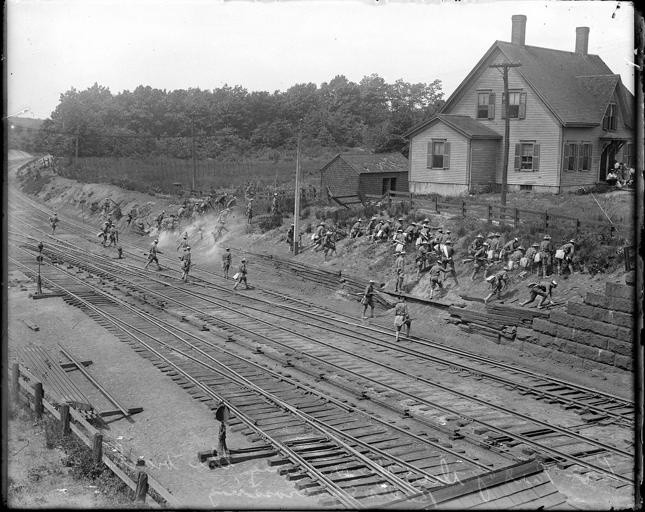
[393,239,407,245]
[398,316,416,327]
[356,293,382,296]
[468,253,493,262]
[417,229,429,240]
[544,301,565,307]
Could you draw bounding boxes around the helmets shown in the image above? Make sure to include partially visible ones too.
[52,192,578,298]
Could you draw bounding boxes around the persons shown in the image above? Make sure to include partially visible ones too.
[143,193,283,290]
[49,213,62,235]
[97,198,119,247]
[606,162,635,190]
[286,184,576,342]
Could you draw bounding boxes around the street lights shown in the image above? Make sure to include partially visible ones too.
[38,241,43,293]
[489,63,522,225]
